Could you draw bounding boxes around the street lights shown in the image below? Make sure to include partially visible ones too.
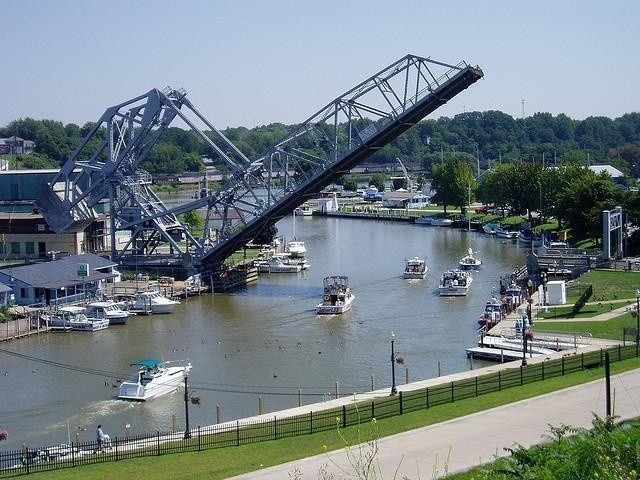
[635,288,640,344]
[527,279,533,324]
[552,258,558,281]
[181,367,195,442]
[521,307,529,367]
[388,329,401,395]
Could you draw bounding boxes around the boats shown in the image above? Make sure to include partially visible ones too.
[295,205,313,216]
[401,248,484,298]
[410,214,572,253]
[314,274,356,316]
[30,286,182,333]
[117,356,192,402]
[252,239,311,275]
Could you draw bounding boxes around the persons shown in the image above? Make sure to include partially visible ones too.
[524,316,530,328]
[519,320,522,331]
[93,424,107,455]
[516,320,520,328]
[441,271,468,288]
[526,305,532,326]
[413,265,419,272]
[526,278,533,295]
